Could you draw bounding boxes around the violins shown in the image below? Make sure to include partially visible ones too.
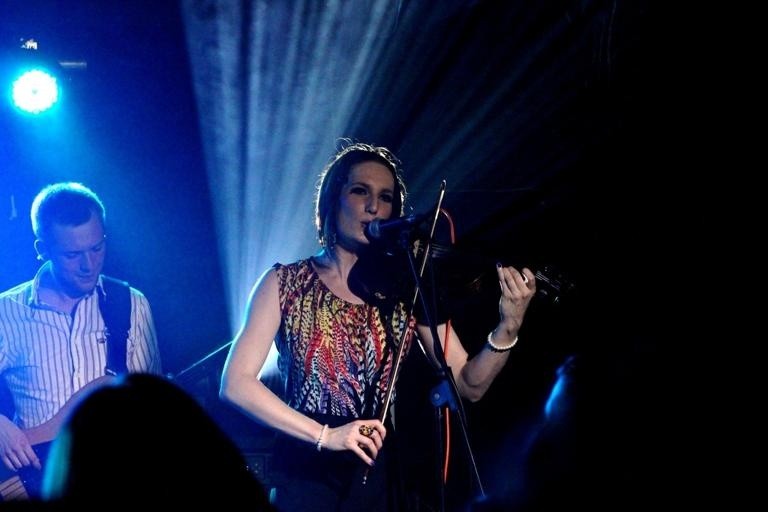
[346,224,573,328]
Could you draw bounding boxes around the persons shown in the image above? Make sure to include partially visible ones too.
[219,137,538,510]
[36,372,275,511]
[1,181,159,500]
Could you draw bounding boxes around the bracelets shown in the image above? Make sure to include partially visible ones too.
[487,326,519,352]
[315,422,328,452]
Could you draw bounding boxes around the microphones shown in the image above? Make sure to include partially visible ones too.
[367,212,420,239]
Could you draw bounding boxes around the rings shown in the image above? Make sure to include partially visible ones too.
[358,423,374,436]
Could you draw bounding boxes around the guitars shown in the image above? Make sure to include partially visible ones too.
[0,340,234,499]
